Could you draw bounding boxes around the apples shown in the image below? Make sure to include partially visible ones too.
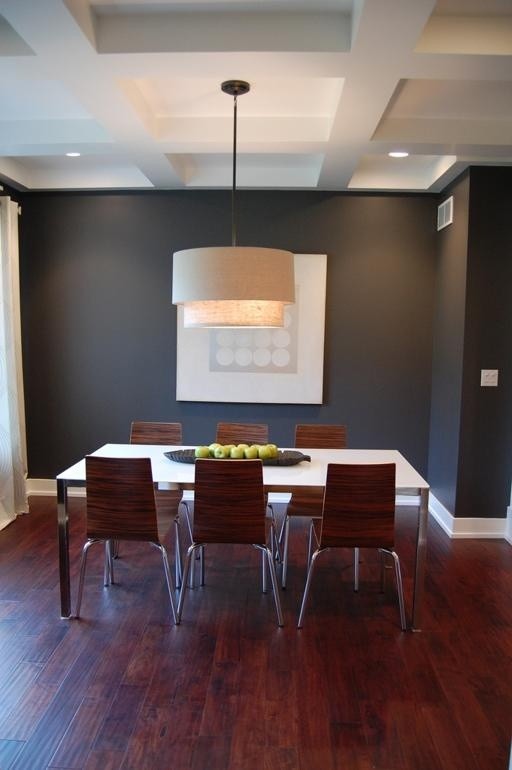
[194,443,277,458]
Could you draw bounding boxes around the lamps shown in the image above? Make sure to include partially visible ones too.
[170,77,299,332]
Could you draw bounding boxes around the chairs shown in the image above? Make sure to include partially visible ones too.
[127,419,184,446]
[294,423,350,451]
[215,421,270,446]
[176,457,285,630]
[295,462,409,632]
[73,453,180,627]
